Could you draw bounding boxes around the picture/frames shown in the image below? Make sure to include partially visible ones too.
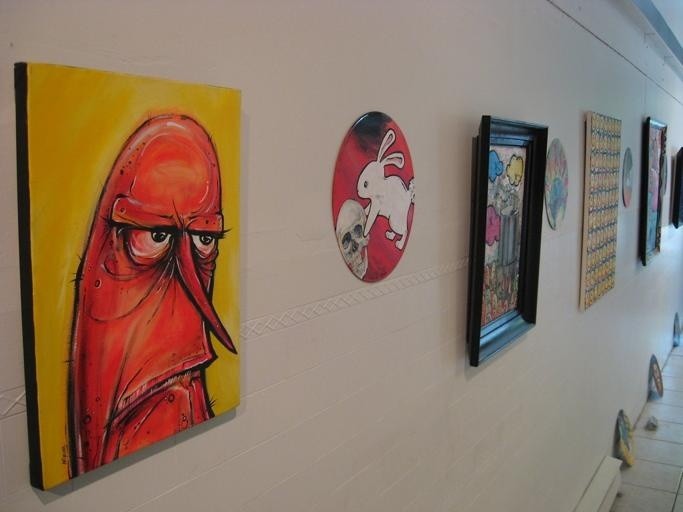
[465,112,550,370]
[640,115,667,267]
